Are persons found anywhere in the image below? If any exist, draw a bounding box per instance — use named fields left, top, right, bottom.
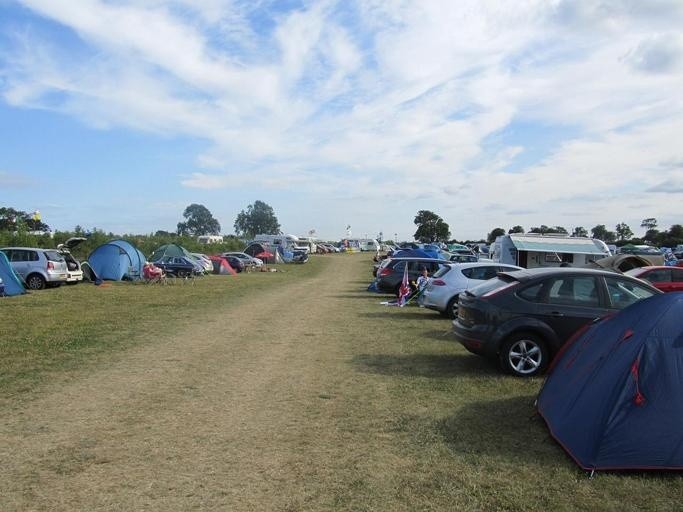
left=413, top=268, right=430, bottom=308
left=144, top=261, right=163, bottom=282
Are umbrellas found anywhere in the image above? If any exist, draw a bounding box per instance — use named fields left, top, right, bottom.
left=255, top=250, right=274, bottom=269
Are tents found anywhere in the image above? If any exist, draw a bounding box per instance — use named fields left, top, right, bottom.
left=533, top=289, right=683, bottom=480
left=368, top=249, right=447, bottom=295
left=209, top=256, right=236, bottom=275
left=87, top=239, right=147, bottom=282
left=0, top=251, right=29, bottom=297
left=147, top=242, right=208, bottom=277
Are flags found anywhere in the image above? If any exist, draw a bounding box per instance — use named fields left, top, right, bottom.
left=380, top=263, right=411, bottom=308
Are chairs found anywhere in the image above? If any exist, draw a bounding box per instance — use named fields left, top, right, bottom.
left=128, top=266, right=160, bottom=286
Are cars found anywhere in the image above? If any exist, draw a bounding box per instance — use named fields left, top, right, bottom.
left=145, top=233, right=340, bottom=279
left=0, top=237, right=88, bottom=289
